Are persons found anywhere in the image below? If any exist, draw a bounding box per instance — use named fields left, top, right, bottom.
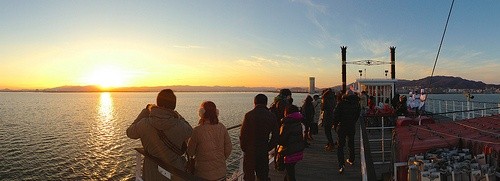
left=420, top=89, right=427, bottom=110
left=187, top=101, right=232, bottom=181
left=414, top=90, right=420, bottom=109
left=312, top=95, right=322, bottom=135
left=320, top=88, right=337, bottom=152
left=300, top=95, right=315, bottom=145
left=277, top=105, right=305, bottom=181
left=268, top=95, right=280, bottom=114
left=126, top=89, right=193, bottom=181
left=333, top=89, right=361, bottom=172
left=358, top=91, right=368, bottom=108
left=368, top=95, right=374, bottom=108
left=335, top=90, right=346, bottom=102
left=409, top=91, right=414, bottom=108
left=271, top=89, right=294, bottom=173
left=240, top=94, right=280, bottom=181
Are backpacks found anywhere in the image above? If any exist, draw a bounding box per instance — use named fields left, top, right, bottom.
left=268, top=98, right=281, bottom=122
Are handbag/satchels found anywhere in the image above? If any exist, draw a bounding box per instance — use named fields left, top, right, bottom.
left=187, top=154, right=195, bottom=175
left=310, top=121, right=318, bottom=134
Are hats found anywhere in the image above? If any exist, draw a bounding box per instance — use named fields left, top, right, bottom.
left=305, top=96, right=313, bottom=101
left=280, top=89, right=292, bottom=97
left=157, top=89, right=176, bottom=107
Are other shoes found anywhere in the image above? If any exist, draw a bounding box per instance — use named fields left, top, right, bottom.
left=274, top=167, right=287, bottom=174
left=346, top=158, right=354, bottom=165
left=324, top=145, right=334, bottom=151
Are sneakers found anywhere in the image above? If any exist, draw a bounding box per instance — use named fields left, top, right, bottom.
left=340, top=168, right=344, bottom=173
